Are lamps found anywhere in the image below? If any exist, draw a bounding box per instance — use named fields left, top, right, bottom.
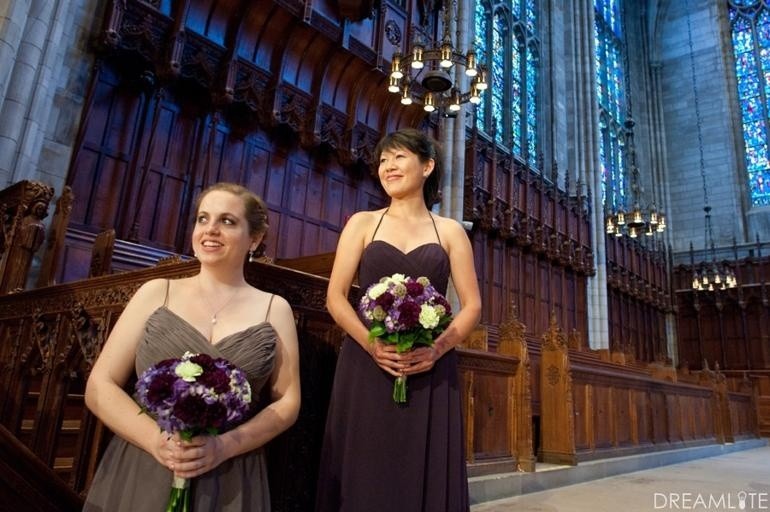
left=388, top=2, right=489, bottom=114
left=603, top=2, right=739, bottom=292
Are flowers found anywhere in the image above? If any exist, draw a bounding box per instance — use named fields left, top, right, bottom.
left=137, top=350, right=252, bottom=511
left=359, top=272, right=450, bottom=405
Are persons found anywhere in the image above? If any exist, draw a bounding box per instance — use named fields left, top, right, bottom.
left=78, top=179, right=304, bottom=510
left=312, top=125, right=486, bottom=512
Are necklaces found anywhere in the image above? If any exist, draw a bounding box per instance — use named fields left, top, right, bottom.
left=194, top=274, right=244, bottom=329
